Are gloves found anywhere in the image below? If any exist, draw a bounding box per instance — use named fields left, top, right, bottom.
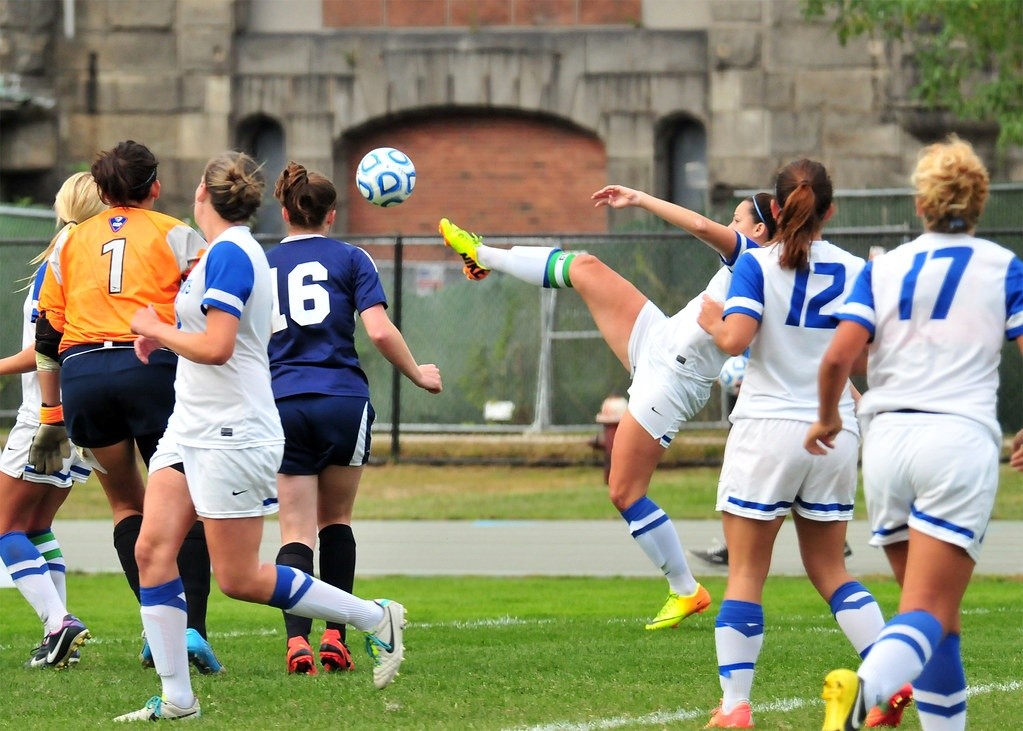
left=28, top=405, right=71, bottom=476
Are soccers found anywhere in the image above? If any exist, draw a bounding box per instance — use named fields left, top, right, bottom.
left=356, top=147, right=417, bottom=208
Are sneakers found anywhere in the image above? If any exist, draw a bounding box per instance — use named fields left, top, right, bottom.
left=138, top=629, right=156, bottom=669
left=29, top=633, right=81, bottom=669
left=285, top=635, right=319, bottom=675
left=818, top=668, right=867, bottom=731
left=703, top=698, right=755, bottom=728
left=319, top=628, right=356, bottom=673
left=44, top=613, right=91, bottom=671
left=437, top=218, right=491, bottom=281
left=362, top=598, right=409, bottom=690
left=113, top=695, right=202, bottom=726
left=865, top=684, right=915, bottom=729
left=645, top=582, right=711, bottom=630
left=186, top=627, right=226, bottom=675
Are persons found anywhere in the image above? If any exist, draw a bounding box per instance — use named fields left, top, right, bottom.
left=439, top=185, right=778, bottom=629
left=697, top=159, right=912, bottom=731
left=802, top=143, right=1023, bottom=731
left=28, top=140, right=225, bottom=675
left=115, top=152, right=410, bottom=722
left=0, top=173, right=110, bottom=672
left=264, top=161, right=442, bottom=674
left=693, top=350, right=862, bottom=564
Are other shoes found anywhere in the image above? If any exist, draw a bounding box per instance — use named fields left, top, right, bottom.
left=694, top=547, right=728, bottom=567
